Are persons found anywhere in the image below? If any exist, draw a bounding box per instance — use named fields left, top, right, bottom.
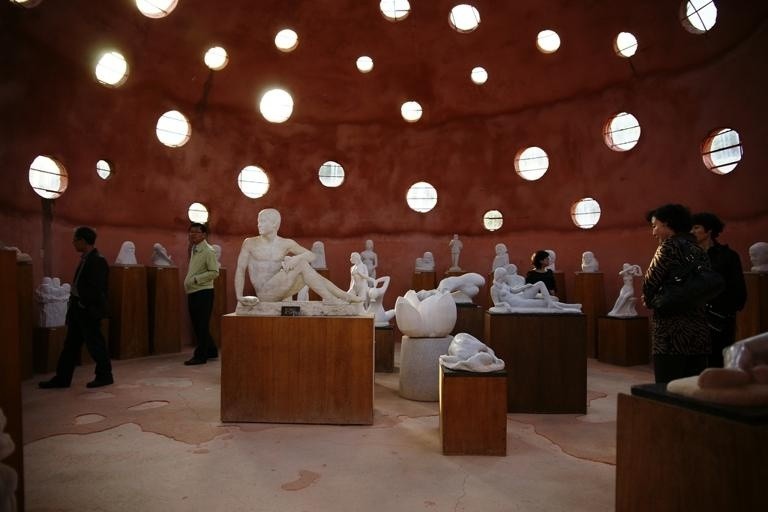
left=38, top=224, right=115, bottom=389
left=416, top=252, right=435, bottom=271
left=607, top=263, right=643, bottom=317
left=234, top=208, right=364, bottom=306
left=545, top=250, right=556, bottom=272
left=749, top=242, right=768, bottom=273
left=491, top=265, right=583, bottom=309
left=448, top=234, right=463, bottom=266
left=151, top=243, right=171, bottom=267
left=34, top=277, right=71, bottom=327
left=525, top=250, right=557, bottom=296
left=184, top=223, right=219, bottom=365
left=493, top=243, right=509, bottom=273
left=643, top=203, right=713, bottom=384
left=311, top=241, right=326, bottom=268
left=366, top=276, right=395, bottom=321
left=115, top=241, right=137, bottom=264
left=360, top=240, right=377, bottom=288
left=581, top=251, right=598, bottom=273
left=697, top=333, right=768, bottom=388
left=347, top=252, right=368, bottom=296
left=688, top=211, right=747, bottom=368
left=211, top=244, right=221, bottom=268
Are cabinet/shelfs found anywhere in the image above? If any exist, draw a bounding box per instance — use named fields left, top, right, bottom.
left=598, top=314, right=651, bottom=366
left=735, top=271, right=768, bottom=340
left=451, top=302, right=484, bottom=343
left=574, top=272, right=607, bottom=358
left=615, top=382, right=768, bottom=511
left=445, top=271, right=465, bottom=293
left=110, top=263, right=148, bottom=360
left=17, top=261, right=34, bottom=380
left=439, top=365, right=509, bottom=458
left=413, top=270, right=436, bottom=291
left=219, top=314, right=374, bottom=425
left=375, top=326, right=395, bottom=373
left=1, top=248, right=26, bottom=512
left=147, top=266, right=182, bottom=354
left=553, top=271, right=567, bottom=303
left=210, top=267, right=228, bottom=329
left=483, top=310, right=589, bottom=415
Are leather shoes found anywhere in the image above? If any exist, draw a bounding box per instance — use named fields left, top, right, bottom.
left=38, top=376, right=71, bottom=388
left=207, top=354, right=218, bottom=361
left=86, top=377, right=113, bottom=388
left=184, top=356, right=206, bottom=365
